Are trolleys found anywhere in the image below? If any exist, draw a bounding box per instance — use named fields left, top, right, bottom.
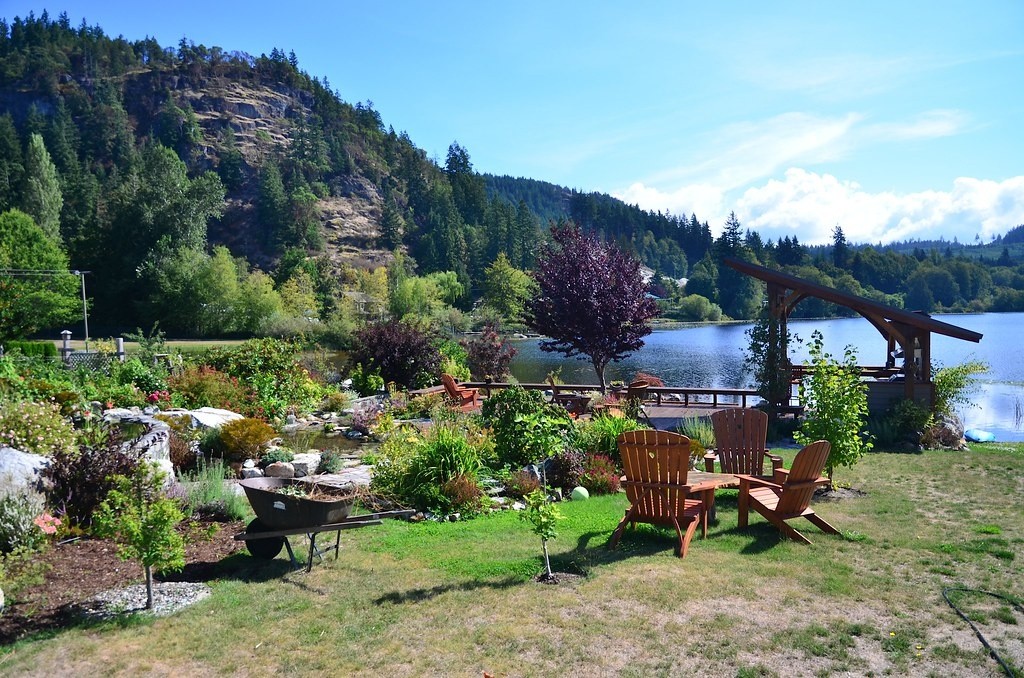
left=234, top=475, right=417, bottom=572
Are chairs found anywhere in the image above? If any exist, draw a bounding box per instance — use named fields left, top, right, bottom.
left=442, top=373, right=480, bottom=407
left=547, top=374, right=580, bottom=416
left=704, top=408, right=783, bottom=519
left=605, top=429, right=708, bottom=559
left=733, top=439, right=843, bottom=547
left=610, top=378, right=650, bottom=420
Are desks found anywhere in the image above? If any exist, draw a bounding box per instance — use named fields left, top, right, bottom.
left=620, top=471, right=756, bottom=518
